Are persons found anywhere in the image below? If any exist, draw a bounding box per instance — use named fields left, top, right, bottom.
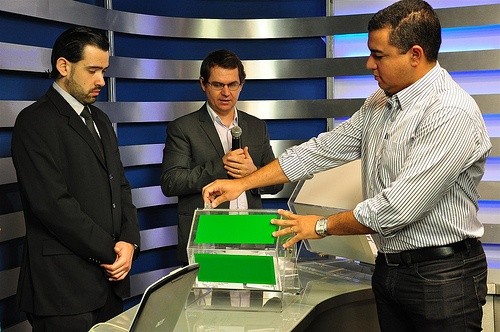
left=200, top=0, right=492, bottom=332
left=161, top=49, right=284, bottom=267
left=11, top=28, right=140, bottom=332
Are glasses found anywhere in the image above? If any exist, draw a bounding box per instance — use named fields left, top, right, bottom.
left=206, top=81, right=240, bottom=91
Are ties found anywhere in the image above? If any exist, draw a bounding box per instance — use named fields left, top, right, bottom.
left=80, top=107, right=104, bottom=162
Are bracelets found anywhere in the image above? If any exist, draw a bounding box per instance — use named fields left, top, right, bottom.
left=132, top=243, right=138, bottom=250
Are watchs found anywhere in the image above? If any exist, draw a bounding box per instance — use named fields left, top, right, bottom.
left=314, top=215, right=336, bottom=238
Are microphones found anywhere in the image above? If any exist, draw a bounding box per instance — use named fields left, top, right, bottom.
left=231, top=126, right=242, bottom=151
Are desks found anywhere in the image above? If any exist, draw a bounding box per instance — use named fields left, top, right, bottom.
left=89, top=259, right=500, bottom=332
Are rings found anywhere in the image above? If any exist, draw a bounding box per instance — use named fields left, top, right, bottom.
left=290, top=225, right=295, bottom=235
left=237, top=169, right=240, bottom=173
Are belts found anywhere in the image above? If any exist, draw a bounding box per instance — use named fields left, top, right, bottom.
left=377, top=238, right=477, bottom=266
